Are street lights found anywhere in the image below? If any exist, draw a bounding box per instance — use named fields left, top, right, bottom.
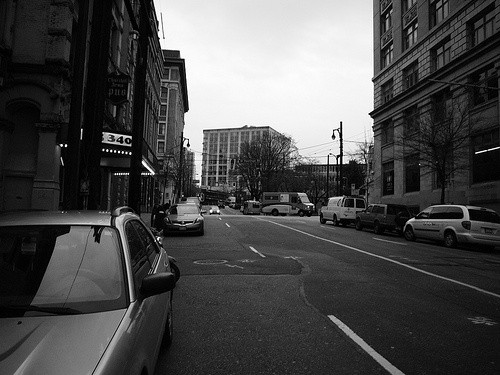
left=178, top=131, right=190, bottom=198
left=332, top=121, right=343, bottom=196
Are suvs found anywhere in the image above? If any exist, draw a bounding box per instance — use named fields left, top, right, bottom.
left=355, top=203, right=420, bottom=237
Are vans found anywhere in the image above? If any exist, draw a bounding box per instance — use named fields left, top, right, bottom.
left=320, top=195, right=368, bottom=227
left=258, top=191, right=316, bottom=217
left=243, top=200, right=261, bottom=216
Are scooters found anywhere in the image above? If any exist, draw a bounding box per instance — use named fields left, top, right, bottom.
left=150, top=225, right=180, bottom=283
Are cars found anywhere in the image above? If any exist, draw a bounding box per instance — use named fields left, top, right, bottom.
left=158, top=202, right=207, bottom=236
left=208, top=205, right=221, bottom=215
left=181, top=196, right=204, bottom=212
left=402, top=204, right=500, bottom=253
left=0, top=205, right=177, bottom=375
